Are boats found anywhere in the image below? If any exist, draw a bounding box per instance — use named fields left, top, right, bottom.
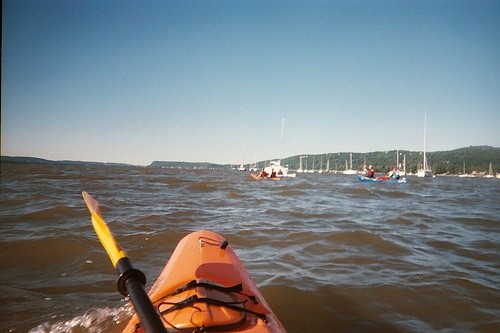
left=250, top=173, right=282, bottom=181
left=356, top=174, right=408, bottom=186
left=460, top=172, right=476, bottom=178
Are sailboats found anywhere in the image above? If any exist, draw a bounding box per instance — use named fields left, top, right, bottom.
left=250, top=154, right=260, bottom=172
left=237, top=139, right=248, bottom=171
left=297, top=151, right=367, bottom=174
left=263, top=116, right=296, bottom=177
left=388, top=150, right=414, bottom=177
left=416, top=113, right=432, bottom=177
left=483, top=160, right=496, bottom=179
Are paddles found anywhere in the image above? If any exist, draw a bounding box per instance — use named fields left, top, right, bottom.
left=81, top=190, right=169, bottom=333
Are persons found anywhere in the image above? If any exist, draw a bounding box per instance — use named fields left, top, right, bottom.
left=278, top=169, right=282, bottom=174
left=390, top=168, right=400, bottom=180
left=365, top=165, right=374, bottom=178
left=270, top=169, right=276, bottom=178
left=260, top=168, right=267, bottom=177
